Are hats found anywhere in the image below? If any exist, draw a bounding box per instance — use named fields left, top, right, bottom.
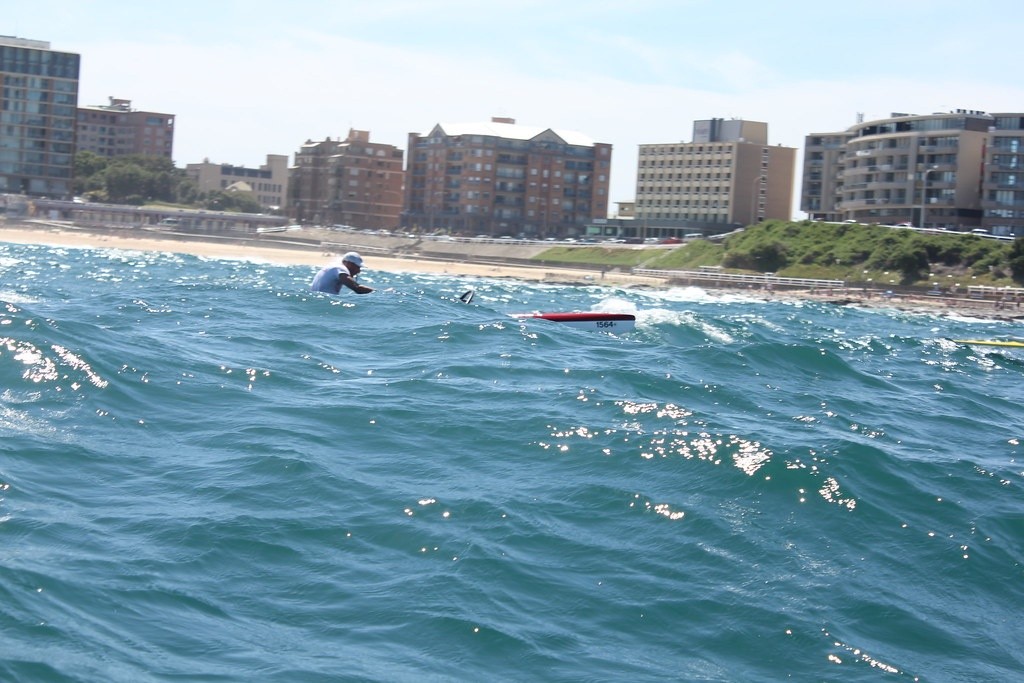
left=342, top=251, right=362, bottom=267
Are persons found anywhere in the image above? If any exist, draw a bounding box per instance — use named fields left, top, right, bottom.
left=780, top=278, right=1023, bottom=312
left=309, top=252, right=373, bottom=297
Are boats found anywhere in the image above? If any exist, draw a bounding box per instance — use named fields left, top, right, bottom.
left=952, top=338, right=1024, bottom=351
left=461, top=292, right=638, bottom=334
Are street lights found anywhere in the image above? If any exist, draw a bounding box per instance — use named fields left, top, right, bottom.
left=919, top=165, right=939, bottom=228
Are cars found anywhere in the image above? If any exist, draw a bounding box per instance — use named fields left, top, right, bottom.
left=810, top=215, right=1017, bottom=241
left=285, top=223, right=704, bottom=246
